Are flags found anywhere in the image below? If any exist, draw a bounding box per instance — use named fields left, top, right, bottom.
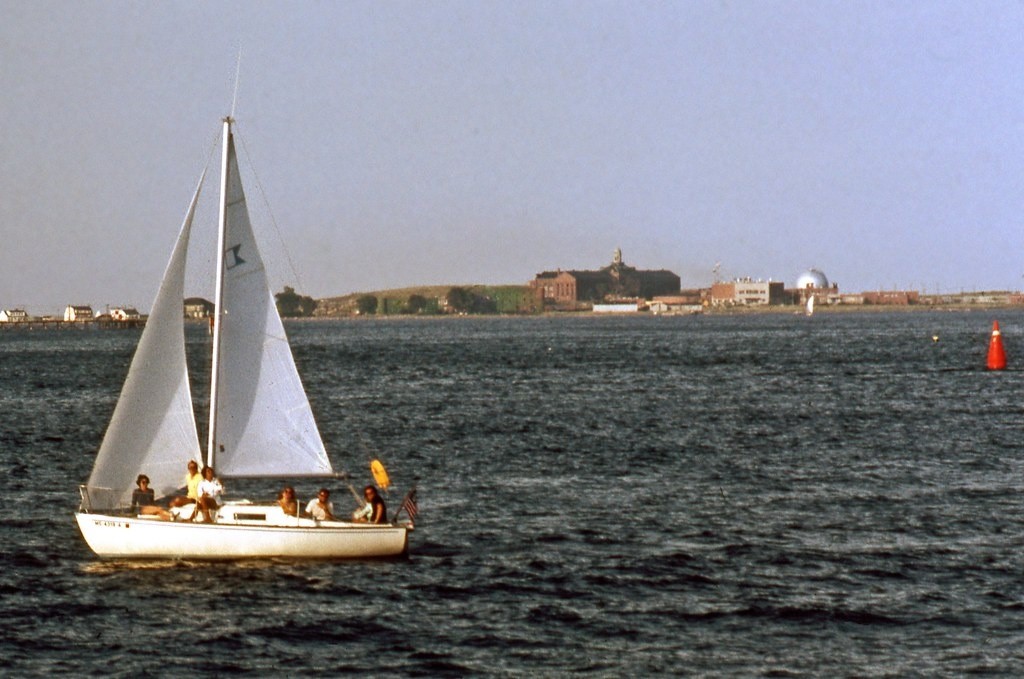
left=404, top=490, right=417, bottom=524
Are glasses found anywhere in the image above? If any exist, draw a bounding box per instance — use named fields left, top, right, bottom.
left=319, top=494, right=328, bottom=498
left=141, top=481, right=147, bottom=484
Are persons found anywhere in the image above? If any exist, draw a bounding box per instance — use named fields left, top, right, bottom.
left=277, top=487, right=297, bottom=515
left=169, top=460, right=204, bottom=507
left=343, top=485, right=387, bottom=524
left=304, top=488, right=338, bottom=520
left=127, top=475, right=180, bottom=522
left=180, top=465, right=224, bottom=523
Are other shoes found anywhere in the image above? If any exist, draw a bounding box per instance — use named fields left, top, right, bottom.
left=202, top=519, right=212, bottom=524
left=174, top=512, right=180, bottom=518
left=169, top=511, right=174, bottom=521
left=182, top=519, right=192, bottom=523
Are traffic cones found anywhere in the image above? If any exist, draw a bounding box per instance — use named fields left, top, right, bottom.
left=987, top=320, right=1008, bottom=371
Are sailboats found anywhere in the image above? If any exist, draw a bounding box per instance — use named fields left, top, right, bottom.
left=68, top=115, right=424, bottom=557
